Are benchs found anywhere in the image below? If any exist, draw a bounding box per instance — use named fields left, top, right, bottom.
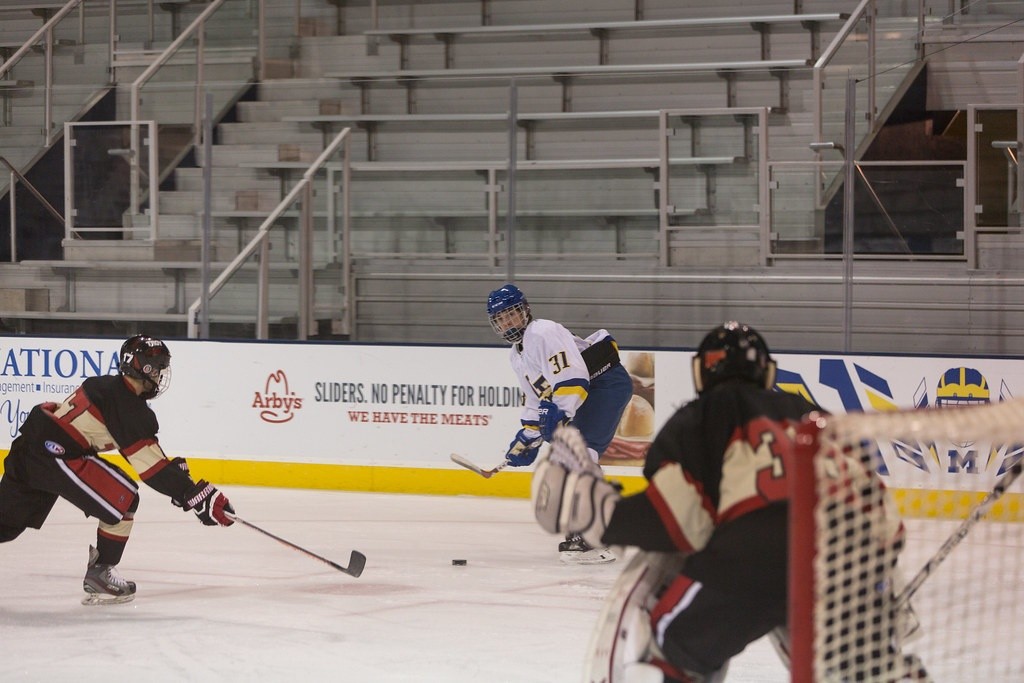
left=0, top=0, right=1024, bottom=356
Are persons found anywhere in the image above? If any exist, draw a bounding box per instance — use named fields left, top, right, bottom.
left=602, top=321, right=930, bottom=683
left=0, top=334, right=235, bottom=605
left=486, top=284, right=633, bottom=564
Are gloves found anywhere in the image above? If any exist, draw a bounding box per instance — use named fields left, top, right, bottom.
left=170, top=456, right=194, bottom=508
left=185, top=478, right=236, bottom=526
left=505, top=428, right=539, bottom=467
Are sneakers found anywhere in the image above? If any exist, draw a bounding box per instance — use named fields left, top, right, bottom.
left=559, top=533, right=616, bottom=567
left=82, top=544, right=136, bottom=605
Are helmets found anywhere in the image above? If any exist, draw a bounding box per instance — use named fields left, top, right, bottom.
left=697, top=321, right=768, bottom=396
left=488, top=283, right=530, bottom=344
left=119, top=335, right=171, bottom=400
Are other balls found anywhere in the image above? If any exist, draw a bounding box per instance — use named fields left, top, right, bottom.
left=451, top=558, right=468, bottom=566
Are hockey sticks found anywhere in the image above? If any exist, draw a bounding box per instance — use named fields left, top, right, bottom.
left=449, top=419, right=568, bottom=480
left=221, top=508, right=367, bottom=579
left=894, top=455, right=1024, bottom=612
